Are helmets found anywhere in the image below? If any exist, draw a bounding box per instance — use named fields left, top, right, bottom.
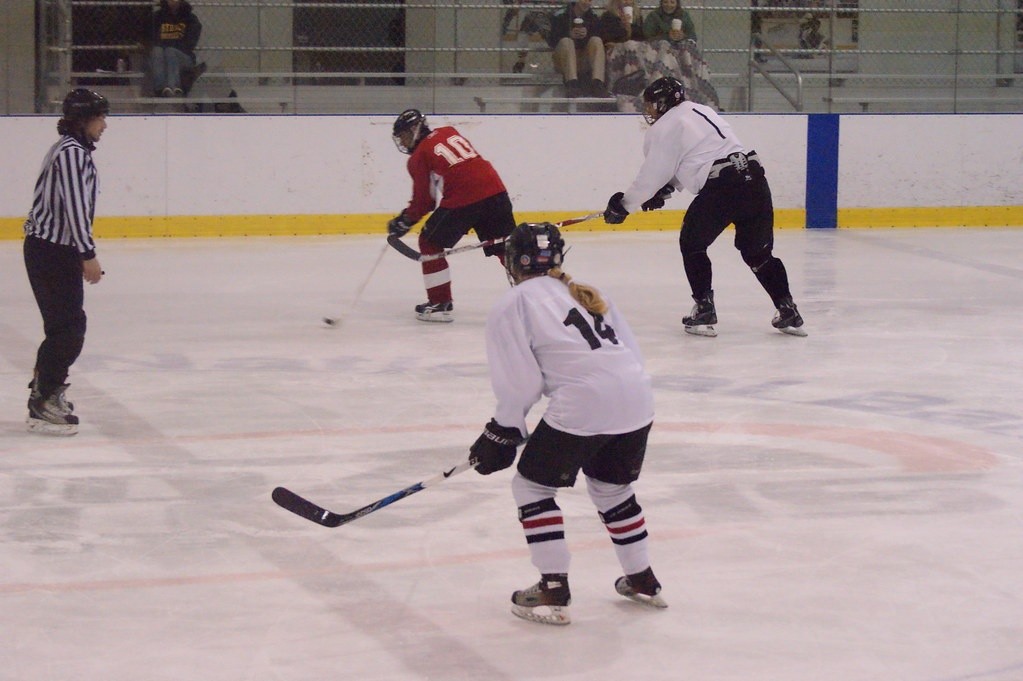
left=61, top=87, right=111, bottom=121
left=504, top=221, right=565, bottom=285
left=642, top=76, right=685, bottom=127
left=391, top=109, right=429, bottom=154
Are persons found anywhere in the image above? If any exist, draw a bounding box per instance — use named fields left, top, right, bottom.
left=21, top=88, right=112, bottom=436
left=467, top=221, right=667, bottom=626
left=144, top=0, right=202, bottom=98
left=379, top=0, right=405, bottom=87
left=604, top=76, right=809, bottom=336
left=293, top=28, right=384, bottom=86
left=116, top=39, right=147, bottom=75
left=499, top=0, right=726, bottom=114
left=751, top=0, right=858, bottom=65
left=384, top=110, right=517, bottom=324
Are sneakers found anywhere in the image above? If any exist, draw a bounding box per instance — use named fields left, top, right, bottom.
left=28, top=368, right=74, bottom=411
left=414, top=297, right=454, bottom=321
left=682, top=290, right=718, bottom=336
left=511, top=572, right=572, bottom=625
left=27, top=378, right=78, bottom=434
left=771, top=295, right=807, bottom=336
left=614, top=568, right=668, bottom=608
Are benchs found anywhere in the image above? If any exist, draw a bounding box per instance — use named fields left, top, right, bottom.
left=44, top=0, right=1023, bottom=112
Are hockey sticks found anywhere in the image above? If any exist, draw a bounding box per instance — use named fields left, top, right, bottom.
left=272, top=432, right=534, bottom=530
left=320, top=242, right=389, bottom=327
left=387, top=194, right=672, bottom=264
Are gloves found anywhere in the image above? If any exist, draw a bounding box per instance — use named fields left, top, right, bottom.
left=641, top=184, right=675, bottom=211
left=468, top=417, right=527, bottom=474
left=604, top=191, right=631, bottom=224
left=387, top=209, right=419, bottom=238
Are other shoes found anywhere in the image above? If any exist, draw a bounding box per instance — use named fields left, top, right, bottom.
left=564, top=78, right=585, bottom=98
left=589, top=80, right=608, bottom=98
left=161, top=87, right=173, bottom=97
left=173, top=87, right=184, bottom=97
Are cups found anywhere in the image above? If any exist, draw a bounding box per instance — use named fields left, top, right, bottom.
left=672, top=19, right=682, bottom=31
left=573, top=18, right=584, bottom=28
left=623, top=6, right=633, bottom=23
